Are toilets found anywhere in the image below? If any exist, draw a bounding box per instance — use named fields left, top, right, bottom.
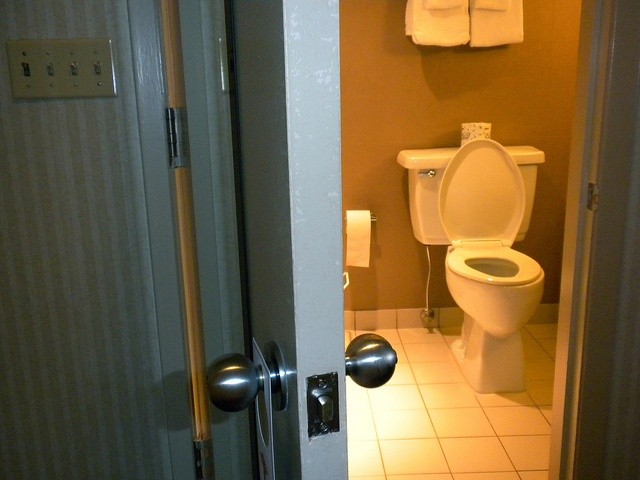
left=397, top=142, right=548, bottom=395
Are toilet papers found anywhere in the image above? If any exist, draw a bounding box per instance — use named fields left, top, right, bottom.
left=346, top=208, right=372, bottom=270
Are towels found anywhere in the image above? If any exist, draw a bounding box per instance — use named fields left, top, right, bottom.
left=470, top=0, right=524, bottom=48
left=405, top=0, right=470, bottom=48
